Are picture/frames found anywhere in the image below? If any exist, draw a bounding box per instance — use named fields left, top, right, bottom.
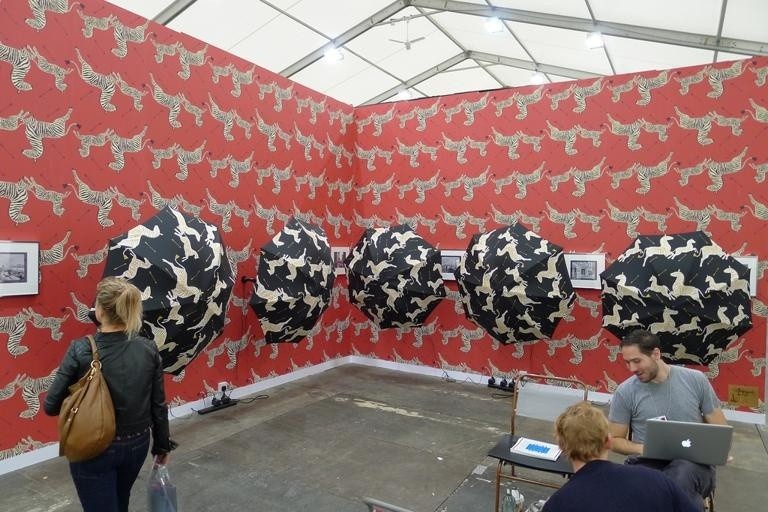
left=330, top=246, right=349, bottom=275
left=438, top=250, right=466, bottom=281
left=732, top=255, right=758, bottom=298
left=562, top=253, right=605, bottom=290
left=0, top=240, right=41, bottom=296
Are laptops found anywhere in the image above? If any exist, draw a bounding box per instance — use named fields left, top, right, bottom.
left=637, top=419, right=734, bottom=469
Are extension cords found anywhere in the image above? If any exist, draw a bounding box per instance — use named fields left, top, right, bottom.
left=487, top=381, right=515, bottom=394
left=197, top=399, right=236, bottom=415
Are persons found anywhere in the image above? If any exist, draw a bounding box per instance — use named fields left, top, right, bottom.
left=606, top=327, right=734, bottom=512
left=538, top=400, right=706, bottom=512
left=42, top=278, right=178, bottom=512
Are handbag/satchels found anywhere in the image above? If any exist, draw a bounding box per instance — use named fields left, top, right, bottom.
left=143, top=453, right=181, bottom=511
left=56, top=327, right=115, bottom=464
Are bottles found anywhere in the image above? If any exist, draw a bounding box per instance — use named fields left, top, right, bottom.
left=501, top=486, right=522, bottom=512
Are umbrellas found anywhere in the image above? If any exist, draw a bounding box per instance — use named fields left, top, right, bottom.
left=452, top=220, right=578, bottom=346
left=86, top=205, right=235, bottom=377
left=596, top=230, right=754, bottom=369
left=244, top=214, right=335, bottom=345
left=342, top=221, right=447, bottom=330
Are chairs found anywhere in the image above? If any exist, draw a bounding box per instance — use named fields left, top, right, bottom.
left=360, top=496, right=412, bottom=512
left=486, top=373, right=588, bottom=512
left=628, top=414, right=715, bottom=512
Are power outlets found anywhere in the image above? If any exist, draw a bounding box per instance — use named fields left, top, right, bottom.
left=218, top=381, right=228, bottom=392
